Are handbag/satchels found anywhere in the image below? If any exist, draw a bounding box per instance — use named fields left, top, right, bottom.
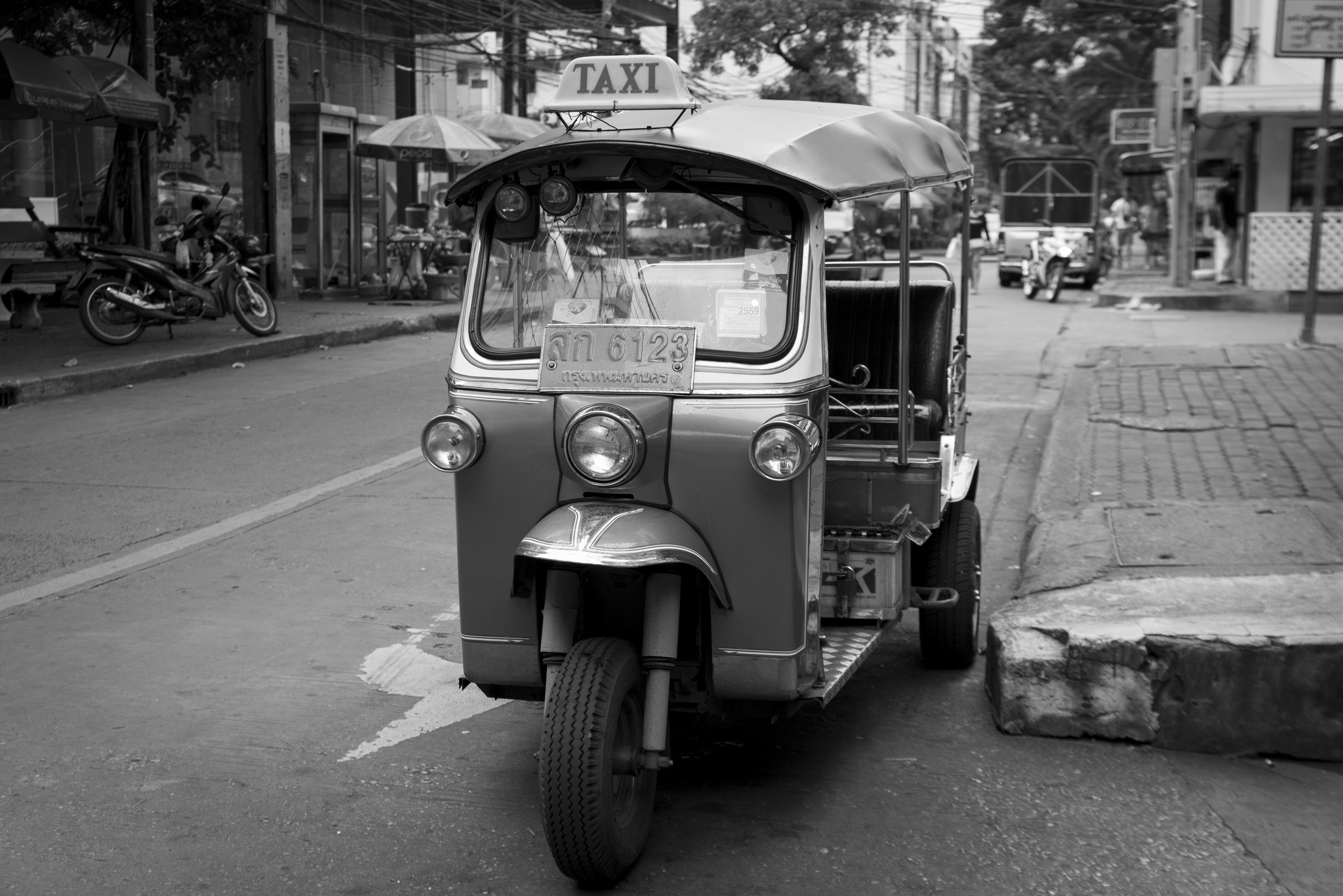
left=174, top=237, right=191, bottom=272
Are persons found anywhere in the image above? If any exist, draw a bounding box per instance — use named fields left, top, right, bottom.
left=958, top=195, right=992, bottom=295
left=1101, top=165, right=1246, bottom=285
left=183, top=193, right=212, bottom=264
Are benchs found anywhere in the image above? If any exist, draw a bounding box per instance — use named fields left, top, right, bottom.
left=823, top=281, right=956, bottom=494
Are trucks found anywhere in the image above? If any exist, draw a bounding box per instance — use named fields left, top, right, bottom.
left=997, top=154, right=1102, bottom=287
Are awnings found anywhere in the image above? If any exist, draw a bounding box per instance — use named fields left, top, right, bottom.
left=1182, top=113, right=1255, bottom=182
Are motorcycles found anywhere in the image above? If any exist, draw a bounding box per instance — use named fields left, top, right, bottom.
left=73, top=181, right=279, bottom=346
left=419, top=53, right=987, bottom=893
left=1018, top=227, right=1076, bottom=303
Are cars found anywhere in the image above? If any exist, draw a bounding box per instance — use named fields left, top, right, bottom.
left=820, top=206, right=889, bottom=285
left=78, top=168, right=244, bottom=236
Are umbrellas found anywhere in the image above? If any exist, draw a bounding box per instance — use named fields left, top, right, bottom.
left=1, top=41, right=171, bottom=247
left=882, top=191, right=934, bottom=212
left=353, top=112, right=499, bottom=236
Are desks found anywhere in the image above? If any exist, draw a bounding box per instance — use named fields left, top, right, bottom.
left=386, top=235, right=462, bottom=300
